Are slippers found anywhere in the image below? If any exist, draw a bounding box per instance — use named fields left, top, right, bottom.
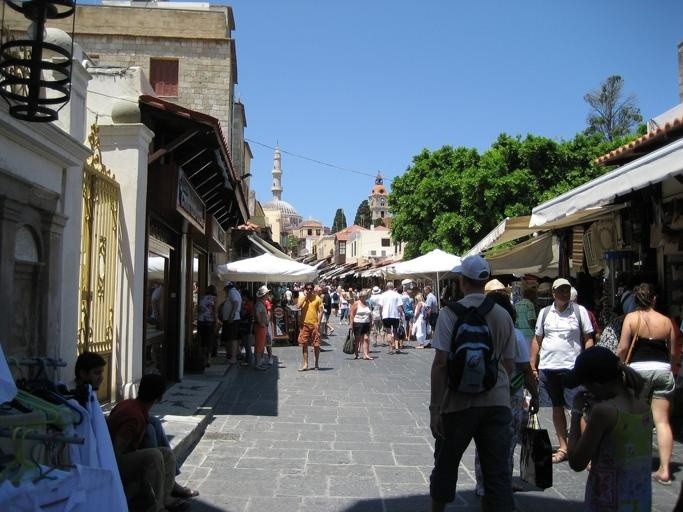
left=170, top=482, right=198, bottom=498
left=553, top=449, right=673, bottom=486
left=165, top=498, right=190, bottom=510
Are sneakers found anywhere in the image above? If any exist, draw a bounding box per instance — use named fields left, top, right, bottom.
left=228, top=357, right=374, bottom=372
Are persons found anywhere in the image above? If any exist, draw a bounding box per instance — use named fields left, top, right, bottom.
left=486, top=279, right=513, bottom=318
left=475, top=292, right=538, bottom=501
left=617, top=283, right=680, bottom=485
left=199, top=281, right=324, bottom=371
left=348, top=278, right=463, bottom=360
left=106, top=374, right=198, bottom=512
left=566, top=286, right=600, bottom=437
left=430, top=256, right=516, bottom=512
left=515, top=283, right=538, bottom=344
left=66, top=351, right=107, bottom=390
left=568, top=347, right=653, bottom=512
left=317, top=281, right=357, bottom=336
left=619, top=275, right=637, bottom=315
left=529, top=278, right=594, bottom=471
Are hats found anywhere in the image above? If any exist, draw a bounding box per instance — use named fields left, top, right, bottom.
left=552, top=278, right=571, bottom=289
left=372, top=286, right=381, bottom=294
left=223, top=280, right=232, bottom=287
left=256, top=285, right=271, bottom=298
left=452, top=255, right=490, bottom=280
left=484, top=279, right=511, bottom=298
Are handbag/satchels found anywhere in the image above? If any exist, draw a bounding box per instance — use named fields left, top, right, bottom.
left=342, top=330, right=355, bottom=354
left=519, top=412, right=552, bottom=489
left=395, top=326, right=407, bottom=340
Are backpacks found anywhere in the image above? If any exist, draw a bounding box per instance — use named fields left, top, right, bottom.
left=423, top=307, right=430, bottom=319
left=441, top=295, right=501, bottom=396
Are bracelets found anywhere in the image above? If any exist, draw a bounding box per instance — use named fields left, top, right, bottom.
left=570, top=408, right=581, bottom=416
left=429, top=405, right=440, bottom=413
